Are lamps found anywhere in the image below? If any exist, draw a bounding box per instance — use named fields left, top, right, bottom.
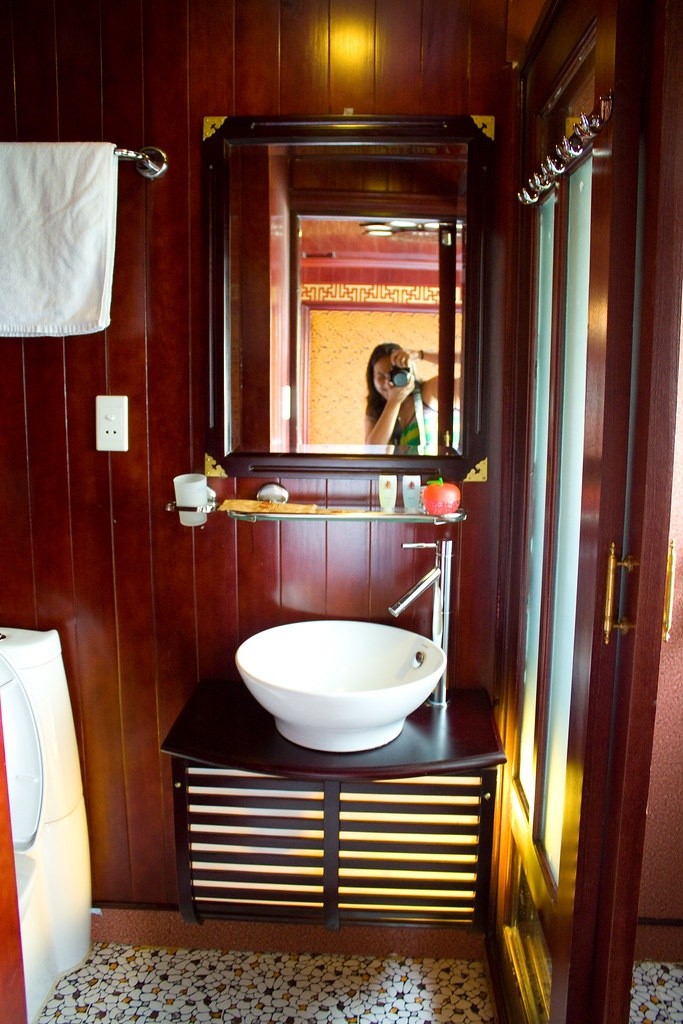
left=358, top=217, right=469, bottom=244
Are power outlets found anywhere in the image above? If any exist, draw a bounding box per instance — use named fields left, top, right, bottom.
left=282, top=386, right=291, bottom=422
left=95, top=394, right=130, bottom=453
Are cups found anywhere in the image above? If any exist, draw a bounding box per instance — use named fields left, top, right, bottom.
left=174, top=473, right=208, bottom=527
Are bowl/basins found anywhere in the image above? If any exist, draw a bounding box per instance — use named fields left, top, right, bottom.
left=235, top=620, right=445, bottom=751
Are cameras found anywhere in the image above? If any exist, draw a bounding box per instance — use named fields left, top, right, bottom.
left=389, top=364, right=411, bottom=387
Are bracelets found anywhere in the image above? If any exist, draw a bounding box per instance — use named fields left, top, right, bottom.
left=421, top=350, right=424, bottom=360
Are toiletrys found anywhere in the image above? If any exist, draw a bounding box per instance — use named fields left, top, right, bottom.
left=379, top=475, right=397, bottom=513
left=402, top=475, right=421, bottom=512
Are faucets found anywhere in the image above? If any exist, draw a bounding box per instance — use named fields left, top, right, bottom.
left=388, top=538, right=452, bottom=708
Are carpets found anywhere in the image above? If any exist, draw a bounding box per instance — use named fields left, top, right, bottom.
left=33, top=940, right=497, bottom=1024
left=625, top=961, right=683, bottom=1024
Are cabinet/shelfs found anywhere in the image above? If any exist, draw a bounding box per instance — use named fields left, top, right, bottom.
left=158, top=678, right=507, bottom=937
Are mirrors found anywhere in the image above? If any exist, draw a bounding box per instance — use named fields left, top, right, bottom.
left=201, top=111, right=491, bottom=484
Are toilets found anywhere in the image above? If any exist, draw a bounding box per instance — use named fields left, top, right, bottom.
left=1, top=627, right=91, bottom=1024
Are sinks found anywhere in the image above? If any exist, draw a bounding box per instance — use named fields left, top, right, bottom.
left=236, top=620, right=445, bottom=753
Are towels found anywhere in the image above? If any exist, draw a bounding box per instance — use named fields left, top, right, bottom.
left=0, top=141, right=118, bottom=337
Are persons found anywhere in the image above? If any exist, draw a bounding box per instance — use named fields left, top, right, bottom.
left=365, top=342, right=461, bottom=455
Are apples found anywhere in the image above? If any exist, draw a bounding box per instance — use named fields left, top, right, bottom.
left=423, top=476, right=461, bottom=516
